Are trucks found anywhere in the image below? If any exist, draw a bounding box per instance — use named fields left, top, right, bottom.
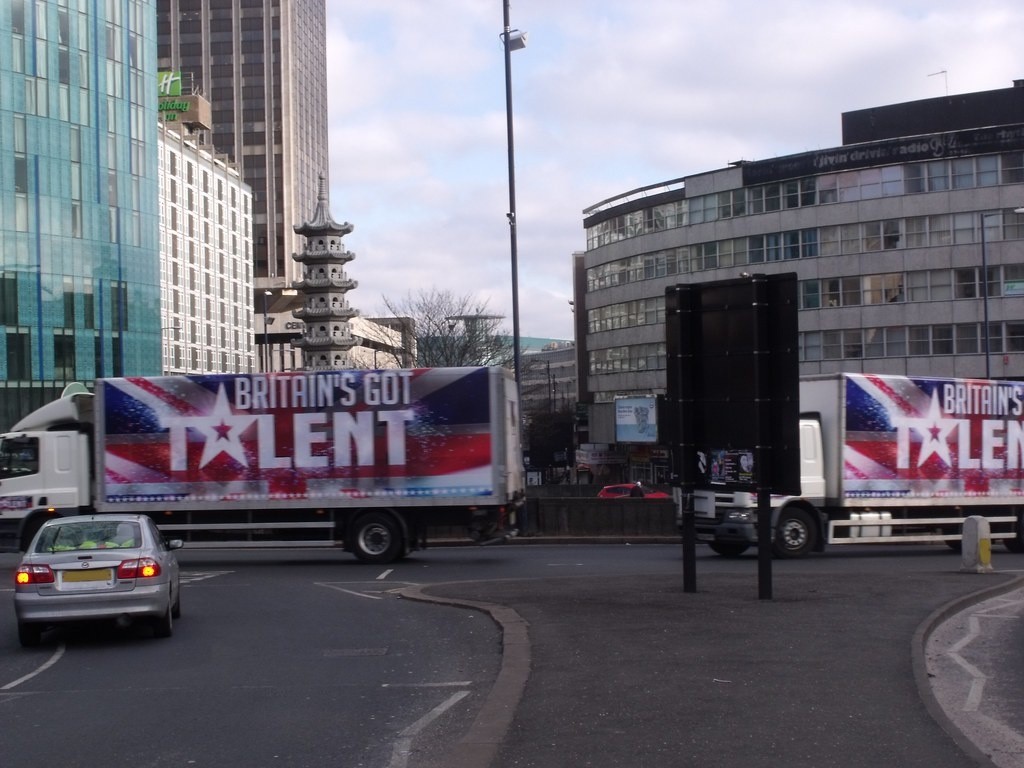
left=0, top=366, right=526, bottom=562
left=672, top=372, right=1024, bottom=555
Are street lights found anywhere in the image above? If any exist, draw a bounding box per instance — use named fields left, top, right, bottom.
left=501, top=26, right=530, bottom=377
left=262, top=291, right=274, bottom=371
left=533, top=359, right=556, bottom=412
left=982, top=208, right=1024, bottom=379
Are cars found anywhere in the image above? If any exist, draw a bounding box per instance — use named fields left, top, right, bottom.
left=597, top=485, right=670, bottom=498
left=14, top=514, right=184, bottom=639
